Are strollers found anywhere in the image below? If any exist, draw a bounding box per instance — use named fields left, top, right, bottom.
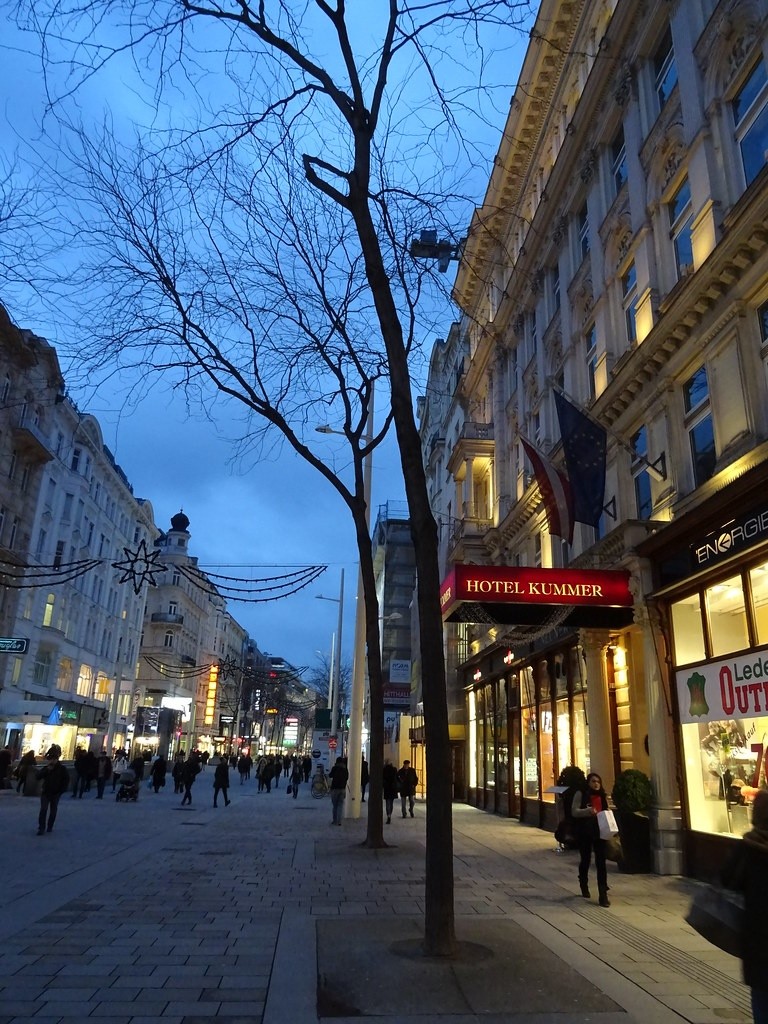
left=115, top=769, right=139, bottom=803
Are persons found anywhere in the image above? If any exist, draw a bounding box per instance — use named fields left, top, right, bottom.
left=71, top=746, right=130, bottom=800
left=397, top=760, right=418, bottom=818
left=172, top=749, right=209, bottom=805
left=34, top=744, right=69, bottom=835
left=255, top=753, right=312, bottom=798
left=384, top=759, right=400, bottom=824
left=238, top=754, right=253, bottom=784
left=150, top=754, right=167, bottom=793
left=329, top=757, right=348, bottom=826
left=0, top=745, right=11, bottom=777
left=213, top=757, right=231, bottom=808
left=129, top=748, right=151, bottom=780
left=741, top=789, right=768, bottom=1024
left=361, top=756, right=369, bottom=801
left=16, top=750, right=37, bottom=793
left=230, top=753, right=238, bottom=770
left=572, top=773, right=611, bottom=905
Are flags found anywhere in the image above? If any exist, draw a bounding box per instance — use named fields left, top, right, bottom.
left=519, top=436, right=574, bottom=547
left=552, top=387, right=608, bottom=526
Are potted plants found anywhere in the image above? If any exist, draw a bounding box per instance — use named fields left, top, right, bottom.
left=556, top=764, right=585, bottom=818
left=611, top=768, right=655, bottom=872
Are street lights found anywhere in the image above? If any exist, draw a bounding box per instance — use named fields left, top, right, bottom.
left=314, top=567, right=346, bottom=788
left=314, top=376, right=375, bottom=822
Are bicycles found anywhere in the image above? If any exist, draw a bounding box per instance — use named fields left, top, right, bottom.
left=311, top=763, right=333, bottom=799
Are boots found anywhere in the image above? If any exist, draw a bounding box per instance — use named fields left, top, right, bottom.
left=579, top=877, right=591, bottom=897
left=599, top=887, right=611, bottom=907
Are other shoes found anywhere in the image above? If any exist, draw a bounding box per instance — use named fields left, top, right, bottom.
left=386, top=817, right=391, bottom=824
left=409, top=810, right=415, bottom=818
left=404, top=812, right=407, bottom=818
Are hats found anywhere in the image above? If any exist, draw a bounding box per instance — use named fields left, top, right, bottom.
left=403, top=760, right=410, bottom=765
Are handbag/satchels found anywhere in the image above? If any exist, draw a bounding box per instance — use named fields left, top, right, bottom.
left=255, top=774, right=261, bottom=778
left=148, top=775, right=153, bottom=790
left=162, top=777, right=165, bottom=787
left=597, top=807, right=619, bottom=840
left=686, top=882, right=745, bottom=959
left=287, top=781, right=292, bottom=794
